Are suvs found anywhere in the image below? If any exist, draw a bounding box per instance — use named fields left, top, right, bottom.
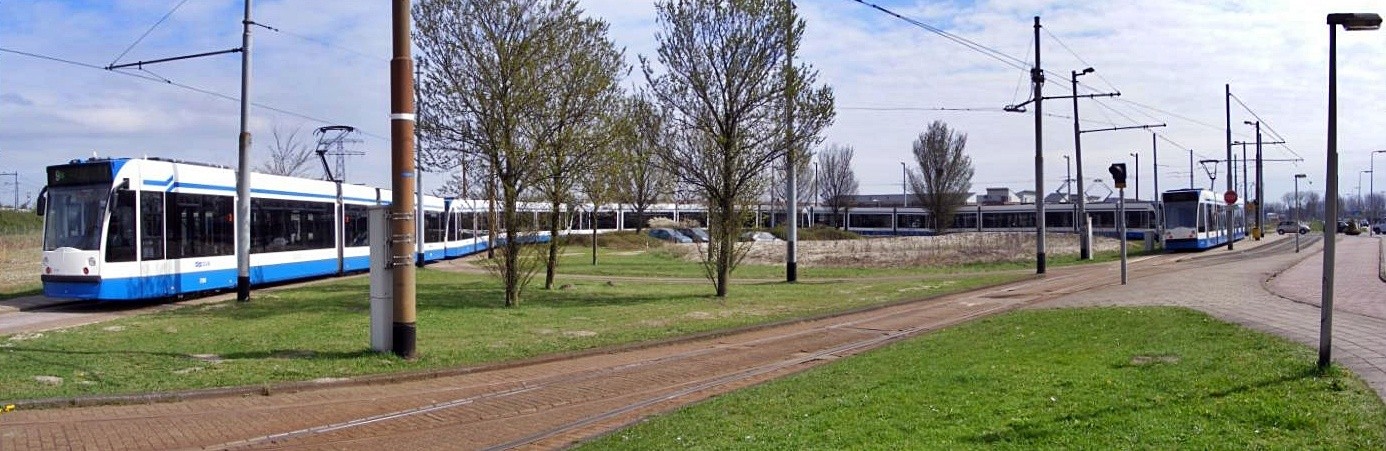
left=1276, top=220, right=1311, bottom=235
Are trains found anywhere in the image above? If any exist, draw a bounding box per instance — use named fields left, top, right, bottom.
left=1159, top=188, right=1246, bottom=252
left=36, top=157, right=1157, bottom=303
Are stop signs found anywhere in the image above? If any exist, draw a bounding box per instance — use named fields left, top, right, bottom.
left=1224, top=191, right=1237, bottom=204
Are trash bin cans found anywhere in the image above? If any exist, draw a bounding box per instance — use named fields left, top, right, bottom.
left=1252, top=228, right=1260, bottom=238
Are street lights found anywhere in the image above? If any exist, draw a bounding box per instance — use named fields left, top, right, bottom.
left=812, top=161, right=818, bottom=206
left=1130, top=152, right=1138, bottom=201
left=1234, top=120, right=1261, bottom=241
left=1316, top=12, right=1383, bottom=369
left=1072, top=67, right=1096, bottom=259
left=900, top=161, right=906, bottom=208
left=1294, top=174, right=1307, bottom=253
left=1063, top=155, right=1071, bottom=204
left=1358, top=150, right=1386, bottom=236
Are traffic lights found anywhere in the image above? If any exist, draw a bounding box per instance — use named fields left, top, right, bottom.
left=1108, top=163, right=1126, bottom=179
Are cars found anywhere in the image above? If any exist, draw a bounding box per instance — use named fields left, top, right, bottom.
left=675, top=227, right=709, bottom=243
left=740, top=231, right=783, bottom=242
left=1321, top=218, right=1369, bottom=233
left=1372, top=217, right=1386, bottom=233
left=647, top=227, right=694, bottom=244
